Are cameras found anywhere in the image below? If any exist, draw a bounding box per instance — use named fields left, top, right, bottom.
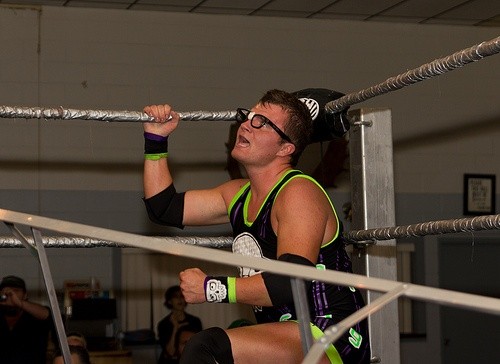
left=0, top=293, right=8, bottom=302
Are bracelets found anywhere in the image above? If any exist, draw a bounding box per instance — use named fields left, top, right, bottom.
left=226, top=277, right=237, bottom=304
left=145, top=131, right=169, bottom=160
left=204, top=276, right=229, bottom=303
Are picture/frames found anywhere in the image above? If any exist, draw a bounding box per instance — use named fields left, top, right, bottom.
left=463, top=173, right=496, bottom=217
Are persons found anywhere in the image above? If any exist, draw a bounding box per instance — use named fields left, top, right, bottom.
left=0, top=275, right=255, bottom=364
left=143, top=89, right=371, bottom=363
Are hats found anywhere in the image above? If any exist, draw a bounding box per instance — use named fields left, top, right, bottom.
left=0, top=276, right=25, bottom=290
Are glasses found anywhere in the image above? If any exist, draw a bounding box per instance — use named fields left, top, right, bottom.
left=236, top=108, right=293, bottom=143
